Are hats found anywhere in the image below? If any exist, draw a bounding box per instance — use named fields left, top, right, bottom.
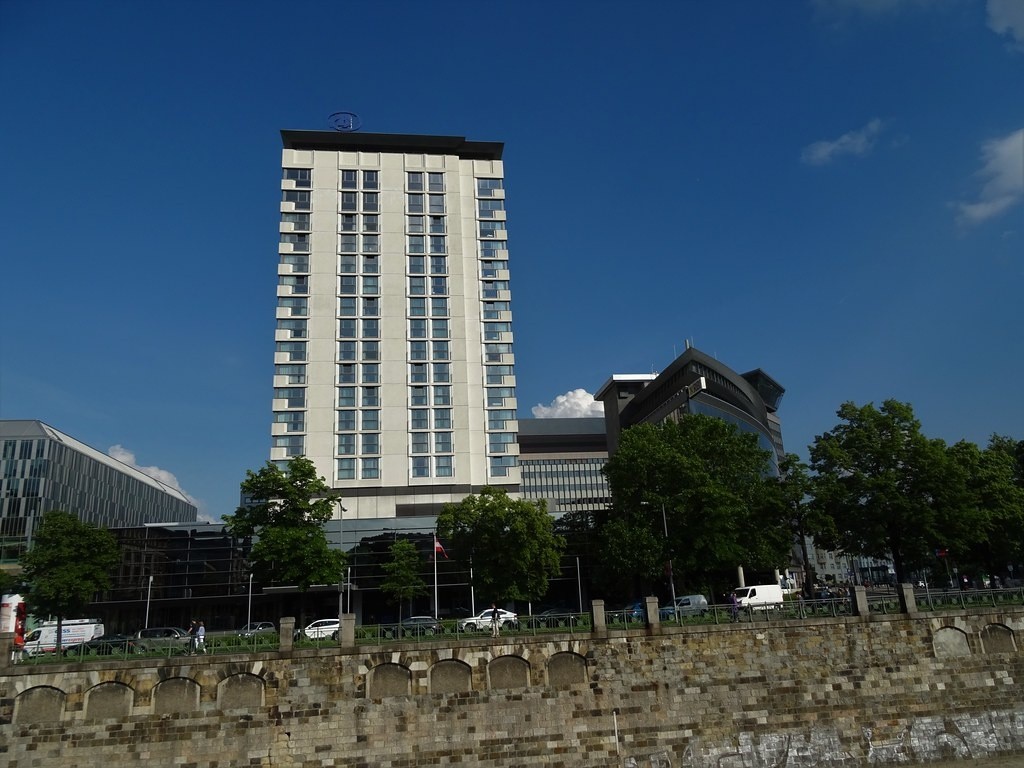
left=199, top=621, right=204, bottom=626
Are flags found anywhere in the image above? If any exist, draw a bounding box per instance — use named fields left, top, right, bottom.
left=436, top=539, right=448, bottom=558
left=931, top=543, right=946, bottom=566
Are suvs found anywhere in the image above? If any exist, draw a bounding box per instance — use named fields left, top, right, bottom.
left=862, top=579, right=876, bottom=587
left=457, top=609, right=518, bottom=632
left=131, top=628, right=207, bottom=653
left=916, top=581, right=929, bottom=589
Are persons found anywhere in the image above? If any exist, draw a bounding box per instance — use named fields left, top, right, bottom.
left=959, top=574, right=968, bottom=591
left=836, top=587, right=850, bottom=615
left=12, top=619, right=26, bottom=664
left=864, top=582, right=869, bottom=587
left=794, top=592, right=808, bottom=618
left=729, top=593, right=739, bottom=623
left=185, top=618, right=207, bottom=656
left=491, top=603, right=500, bottom=638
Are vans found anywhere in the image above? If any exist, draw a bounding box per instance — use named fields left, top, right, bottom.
left=12, top=619, right=104, bottom=656
left=660, top=594, right=709, bottom=619
left=731, top=585, right=784, bottom=612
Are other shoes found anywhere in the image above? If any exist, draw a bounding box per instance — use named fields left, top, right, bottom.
left=21, top=660, right=24, bottom=663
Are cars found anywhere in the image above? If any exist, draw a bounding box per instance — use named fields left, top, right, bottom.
left=527, top=608, right=580, bottom=629
left=383, top=617, right=445, bottom=638
left=294, top=619, right=340, bottom=643
left=620, top=602, right=678, bottom=623
left=805, top=588, right=841, bottom=608
left=238, top=622, right=276, bottom=638
left=61, top=633, right=137, bottom=654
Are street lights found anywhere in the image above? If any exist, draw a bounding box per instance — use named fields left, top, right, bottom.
left=336, top=495, right=348, bottom=615
left=249, top=574, right=254, bottom=633
left=145, top=575, right=154, bottom=627
